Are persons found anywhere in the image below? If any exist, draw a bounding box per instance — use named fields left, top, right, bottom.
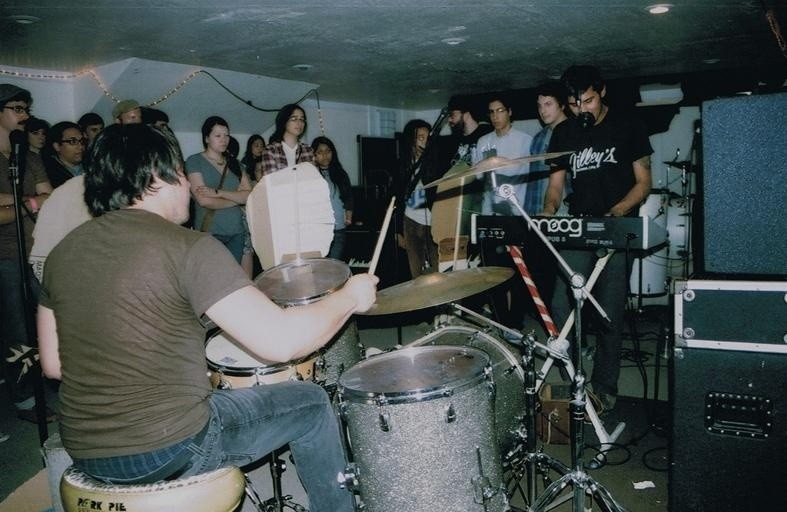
left=37, top=126, right=379, bottom=512
left=390, top=66, right=653, bottom=411
left=1, top=84, right=353, bottom=440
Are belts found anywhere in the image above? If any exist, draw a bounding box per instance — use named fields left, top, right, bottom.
left=86, top=415, right=211, bottom=485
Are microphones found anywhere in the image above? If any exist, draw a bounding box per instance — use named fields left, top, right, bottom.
left=10, top=130, right=26, bottom=183
left=496, top=183, right=520, bottom=206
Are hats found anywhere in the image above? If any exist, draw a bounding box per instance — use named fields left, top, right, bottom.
left=0, top=83, right=30, bottom=103
left=112, top=99, right=147, bottom=121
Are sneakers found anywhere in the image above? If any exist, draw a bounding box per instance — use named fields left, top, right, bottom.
left=14, top=404, right=59, bottom=426
left=501, top=326, right=525, bottom=344
left=582, top=388, right=619, bottom=426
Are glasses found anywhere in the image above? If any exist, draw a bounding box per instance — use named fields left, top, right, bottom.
left=59, top=138, right=86, bottom=146
left=289, top=116, right=305, bottom=122
left=1, top=105, right=29, bottom=113
left=487, top=109, right=509, bottom=115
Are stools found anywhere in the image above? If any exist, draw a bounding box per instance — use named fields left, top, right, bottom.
left=59, top=465, right=247, bottom=511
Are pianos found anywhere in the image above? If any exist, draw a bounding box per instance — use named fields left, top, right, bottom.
left=470, top=211, right=669, bottom=250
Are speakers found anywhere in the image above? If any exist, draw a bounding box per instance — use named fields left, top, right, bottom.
left=670, top=349, right=787, bottom=512
left=429, top=107, right=449, bottom=138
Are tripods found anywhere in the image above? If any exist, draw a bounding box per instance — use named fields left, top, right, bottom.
left=452, top=288, right=631, bottom=512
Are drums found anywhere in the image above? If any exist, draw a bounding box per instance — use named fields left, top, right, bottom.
left=204, top=329, right=316, bottom=390
left=255, top=258, right=362, bottom=390
left=337, top=326, right=526, bottom=510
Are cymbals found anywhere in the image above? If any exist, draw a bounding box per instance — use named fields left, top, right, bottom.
left=362, top=265, right=516, bottom=317
left=422, top=151, right=573, bottom=189
